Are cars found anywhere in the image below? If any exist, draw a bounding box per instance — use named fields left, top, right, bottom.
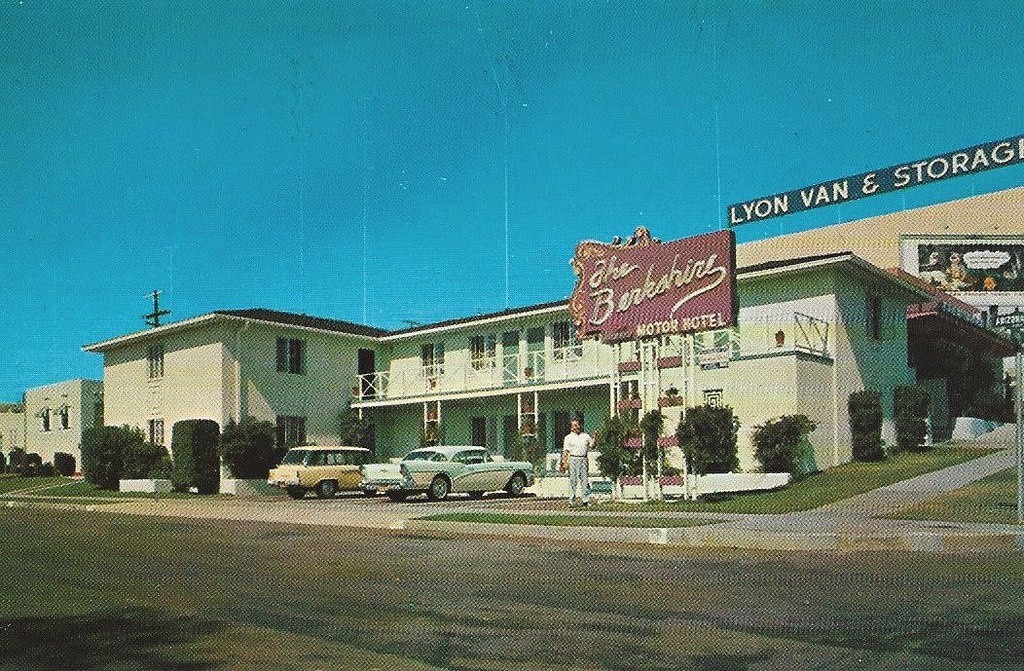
left=360, top=444, right=536, bottom=501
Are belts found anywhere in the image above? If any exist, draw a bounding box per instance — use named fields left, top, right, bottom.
left=570, top=454, right=587, bottom=457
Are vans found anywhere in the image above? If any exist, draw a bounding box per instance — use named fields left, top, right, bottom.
left=268, top=445, right=379, bottom=498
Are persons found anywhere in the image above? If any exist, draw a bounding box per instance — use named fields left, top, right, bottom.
left=561, top=417, right=597, bottom=509
left=921, top=250, right=968, bottom=291
